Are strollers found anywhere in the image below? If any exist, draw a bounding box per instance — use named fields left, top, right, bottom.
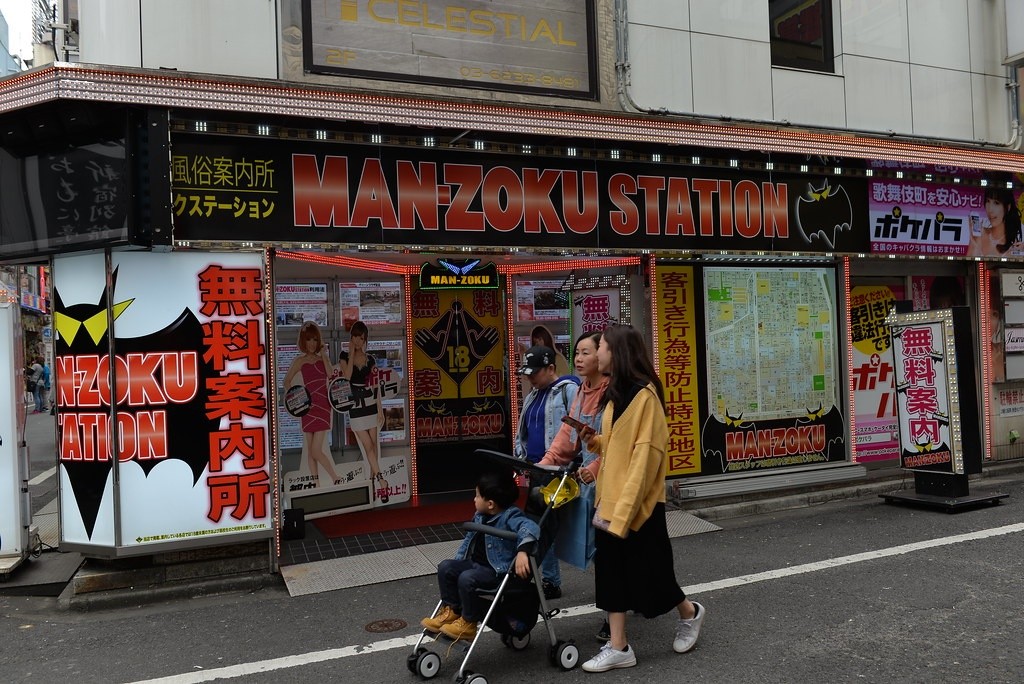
left=407, top=448, right=582, bottom=684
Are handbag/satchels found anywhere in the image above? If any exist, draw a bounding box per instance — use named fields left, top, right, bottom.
left=554, top=469, right=597, bottom=570
left=27, top=381, right=36, bottom=391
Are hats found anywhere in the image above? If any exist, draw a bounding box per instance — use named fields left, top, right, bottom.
left=514, top=346, right=555, bottom=375
left=541, top=476, right=579, bottom=508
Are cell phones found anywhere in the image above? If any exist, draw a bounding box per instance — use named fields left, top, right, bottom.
left=560, top=415, right=596, bottom=438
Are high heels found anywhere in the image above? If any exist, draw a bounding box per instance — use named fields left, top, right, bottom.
left=372, top=478, right=375, bottom=502
left=381, top=481, right=388, bottom=503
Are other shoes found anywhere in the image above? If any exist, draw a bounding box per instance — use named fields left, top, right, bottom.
left=30, top=410, right=42, bottom=414
left=596, top=618, right=611, bottom=640
left=43, top=407, right=48, bottom=410
left=540, top=584, right=562, bottom=600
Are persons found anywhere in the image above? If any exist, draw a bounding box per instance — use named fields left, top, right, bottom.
left=283, top=321, right=338, bottom=489
left=513, top=346, right=578, bottom=598
left=965, top=186, right=1024, bottom=257
left=338, top=321, right=389, bottom=503
left=577, top=324, right=705, bottom=671
left=419, top=475, right=540, bottom=642
left=536, top=331, right=611, bottom=640
left=519, top=326, right=569, bottom=377
left=28, top=357, right=50, bottom=414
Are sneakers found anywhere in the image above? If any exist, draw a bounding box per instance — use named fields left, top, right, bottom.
left=581, top=640, right=637, bottom=672
left=432, top=616, right=477, bottom=662
left=672, top=601, right=705, bottom=652
left=419, top=606, right=462, bottom=633
